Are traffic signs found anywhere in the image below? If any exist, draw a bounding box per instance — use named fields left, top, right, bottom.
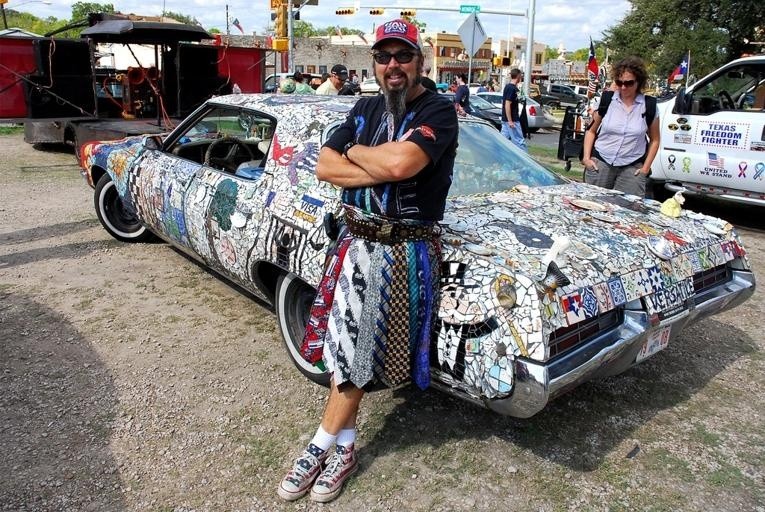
left=460, top=5, right=481, bottom=15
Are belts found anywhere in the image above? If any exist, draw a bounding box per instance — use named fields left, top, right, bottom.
left=343, top=209, right=436, bottom=243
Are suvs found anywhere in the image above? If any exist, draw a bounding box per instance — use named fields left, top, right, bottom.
left=558, top=53, right=765, bottom=207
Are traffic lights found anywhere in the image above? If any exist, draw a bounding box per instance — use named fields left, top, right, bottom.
left=336, top=8, right=355, bottom=14
left=492, top=57, right=511, bottom=68
left=370, top=9, right=384, bottom=15
left=401, top=9, right=416, bottom=16
left=270, top=5, right=288, bottom=39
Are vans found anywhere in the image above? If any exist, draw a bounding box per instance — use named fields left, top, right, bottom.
left=265, top=73, right=323, bottom=94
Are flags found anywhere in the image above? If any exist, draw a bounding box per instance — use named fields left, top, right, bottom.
left=588, top=38, right=599, bottom=93
left=668, top=52, right=689, bottom=84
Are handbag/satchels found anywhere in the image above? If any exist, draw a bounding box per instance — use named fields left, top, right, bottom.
left=461, top=94, right=467, bottom=103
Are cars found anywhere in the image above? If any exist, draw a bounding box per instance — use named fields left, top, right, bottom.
left=80, top=94, right=755, bottom=418
left=338, top=79, right=381, bottom=95
left=440, top=84, right=588, bottom=132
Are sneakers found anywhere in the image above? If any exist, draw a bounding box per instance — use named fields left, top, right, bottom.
left=311, top=446, right=359, bottom=503
left=278, top=444, right=329, bottom=501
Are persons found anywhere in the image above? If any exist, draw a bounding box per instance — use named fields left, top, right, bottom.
left=419, top=64, right=437, bottom=93
left=581, top=55, right=660, bottom=200
left=276, top=18, right=459, bottom=503
left=476, top=78, right=501, bottom=93
left=675, top=79, right=686, bottom=95
left=280, top=63, right=368, bottom=95
left=454, top=71, right=469, bottom=116
left=499, top=69, right=528, bottom=153
left=448, top=80, right=459, bottom=94
left=652, top=78, right=660, bottom=97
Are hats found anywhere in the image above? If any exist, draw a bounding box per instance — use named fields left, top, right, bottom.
left=331, top=65, right=349, bottom=80
left=371, top=19, right=423, bottom=49
left=509, top=68, right=520, bottom=75
left=280, top=79, right=296, bottom=93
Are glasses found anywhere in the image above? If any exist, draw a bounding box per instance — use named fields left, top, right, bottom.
left=373, top=51, right=418, bottom=63
left=614, top=79, right=636, bottom=87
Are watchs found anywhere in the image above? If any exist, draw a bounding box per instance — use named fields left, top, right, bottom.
left=342, top=141, right=358, bottom=157
